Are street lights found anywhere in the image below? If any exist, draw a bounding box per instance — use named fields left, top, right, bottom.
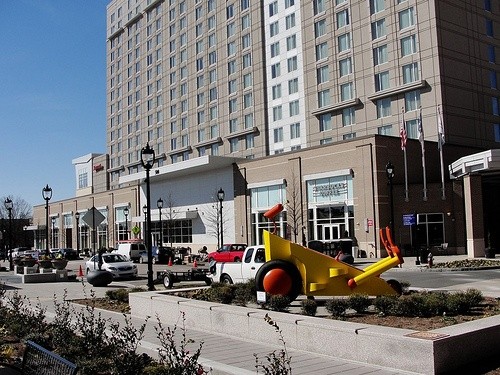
left=385, top=161, right=396, bottom=243
left=157, top=198, right=163, bottom=248
left=41, top=184, right=53, bottom=255
left=4, top=198, right=14, bottom=271
left=217, top=188, right=224, bottom=247
left=143, top=205, right=148, bottom=247
left=237, top=167, right=248, bottom=245
left=52, top=217, right=56, bottom=248
left=75, top=212, right=80, bottom=259
left=289, top=156, right=307, bottom=247
left=124, top=207, right=129, bottom=239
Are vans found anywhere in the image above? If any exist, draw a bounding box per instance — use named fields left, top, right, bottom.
left=111, top=243, right=146, bottom=262
left=140, top=142, right=156, bottom=291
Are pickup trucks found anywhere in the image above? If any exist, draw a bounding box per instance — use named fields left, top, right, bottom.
left=207, top=245, right=266, bottom=286
left=206, top=244, right=247, bottom=263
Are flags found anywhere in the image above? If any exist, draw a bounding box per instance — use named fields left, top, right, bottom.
left=400, top=109, right=407, bottom=150
left=418, top=108, right=425, bottom=145
left=437, top=107, right=445, bottom=149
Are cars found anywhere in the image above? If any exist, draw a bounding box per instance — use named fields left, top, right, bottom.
left=7, top=247, right=77, bottom=260
left=139, top=246, right=173, bottom=265
left=85, top=252, right=138, bottom=280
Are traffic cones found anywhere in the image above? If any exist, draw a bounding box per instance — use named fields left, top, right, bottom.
left=193, top=257, right=197, bottom=268
left=167, top=256, right=173, bottom=266
left=78, top=265, right=84, bottom=276
